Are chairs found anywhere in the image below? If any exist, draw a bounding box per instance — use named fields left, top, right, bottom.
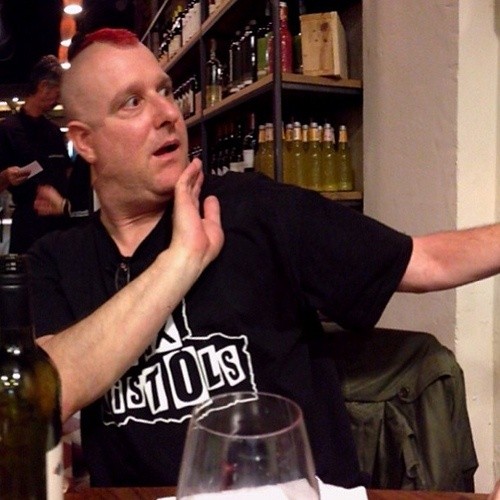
left=341, top=327, right=479, bottom=494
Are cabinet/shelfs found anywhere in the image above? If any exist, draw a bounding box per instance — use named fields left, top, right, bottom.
left=140, top=0, right=365, bottom=200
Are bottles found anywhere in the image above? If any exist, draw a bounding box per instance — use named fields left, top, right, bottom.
left=0, top=254, right=63, bottom=500
left=172, top=2, right=302, bottom=119
left=188, top=117, right=353, bottom=192
left=156, top=0, right=228, bottom=67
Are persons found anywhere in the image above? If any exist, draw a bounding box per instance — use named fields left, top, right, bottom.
left=0, top=18, right=500, bottom=486
left=1, top=55, right=101, bottom=254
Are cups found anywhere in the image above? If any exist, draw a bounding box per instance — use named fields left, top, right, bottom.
left=176, top=392, right=320, bottom=500
